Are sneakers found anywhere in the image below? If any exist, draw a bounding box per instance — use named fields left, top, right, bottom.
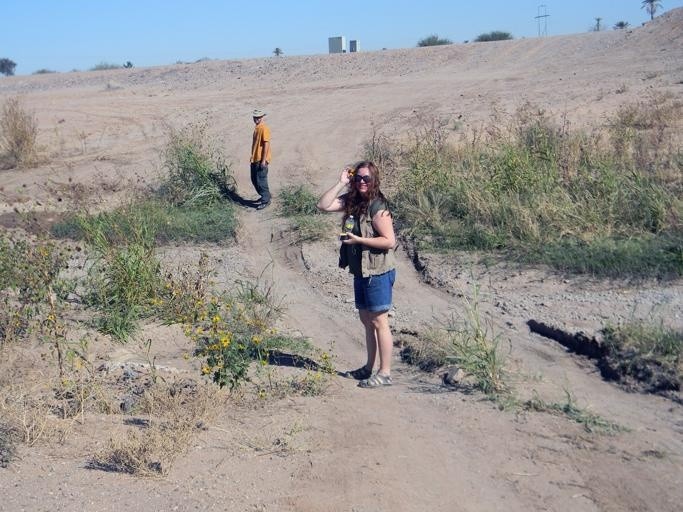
left=358, top=371, right=393, bottom=389
left=345, top=365, right=380, bottom=380
left=252, top=199, right=271, bottom=210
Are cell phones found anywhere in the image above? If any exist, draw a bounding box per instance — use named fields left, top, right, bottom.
left=338, top=233, right=353, bottom=240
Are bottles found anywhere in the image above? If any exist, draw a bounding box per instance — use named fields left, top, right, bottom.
left=342, top=214, right=354, bottom=233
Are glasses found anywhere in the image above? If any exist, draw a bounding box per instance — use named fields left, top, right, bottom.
left=354, top=175, right=370, bottom=183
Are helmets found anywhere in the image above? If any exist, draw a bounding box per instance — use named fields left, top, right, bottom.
left=252, top=109, right=266, bottom=118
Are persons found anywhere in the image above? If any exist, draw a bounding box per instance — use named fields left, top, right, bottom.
left=316, top=161, right=396, bottom=388
left=250, top=110, right=272, bottom=210
